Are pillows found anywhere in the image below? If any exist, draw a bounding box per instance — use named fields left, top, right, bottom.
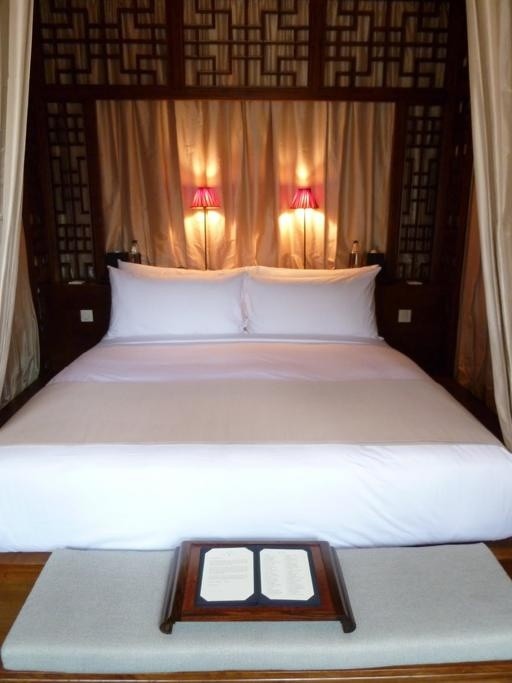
left=101, top=251, right=381, bottom=344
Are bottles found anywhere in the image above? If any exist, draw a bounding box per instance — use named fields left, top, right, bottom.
left=349, top=239, right=361, bottom=269
left=130, top=240, right=142, bottom=265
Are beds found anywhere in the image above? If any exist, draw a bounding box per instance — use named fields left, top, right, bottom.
left=1, top=254, right=512, bottom=552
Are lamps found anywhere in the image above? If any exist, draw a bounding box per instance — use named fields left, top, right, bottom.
left=192, top=186, right=222, bottom=271
left=289, top=187, right=319, bottom=270
